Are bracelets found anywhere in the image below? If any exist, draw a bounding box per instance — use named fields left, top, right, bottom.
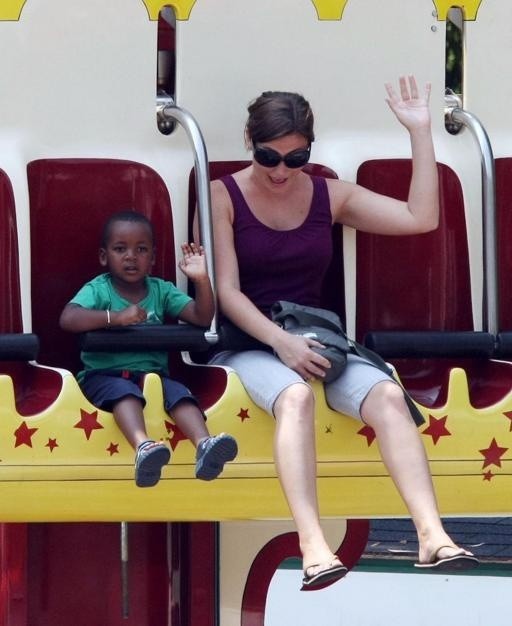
left=107, top=309, right=111, bottom=327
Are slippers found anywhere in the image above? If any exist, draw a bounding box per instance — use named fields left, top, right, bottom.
left=415, top=545, right=479, bottom=571
left=304, top=556, right=347, bottom=586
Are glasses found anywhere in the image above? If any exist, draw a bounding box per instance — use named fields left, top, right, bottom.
left=252, top=139, right=312, bottom=167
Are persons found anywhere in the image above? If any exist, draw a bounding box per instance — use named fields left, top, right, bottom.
left=192, top=74, right=484, bottom=591
left=58, top=210, right=239, bottom=488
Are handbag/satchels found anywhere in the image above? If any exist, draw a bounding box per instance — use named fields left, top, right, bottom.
left=273, top=301, right=349, bottom=382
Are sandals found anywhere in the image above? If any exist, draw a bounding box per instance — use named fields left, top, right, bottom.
left=195, top=435, right=237, bottom=480
left=134, top=440, right=170, bottom=487
